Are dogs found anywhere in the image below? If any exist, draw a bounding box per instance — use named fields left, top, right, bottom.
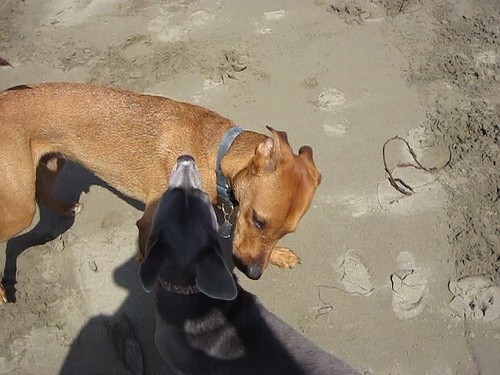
left=137, top=155, right=361, bottom=374
left=0, top=58, right=322, bottom=306
